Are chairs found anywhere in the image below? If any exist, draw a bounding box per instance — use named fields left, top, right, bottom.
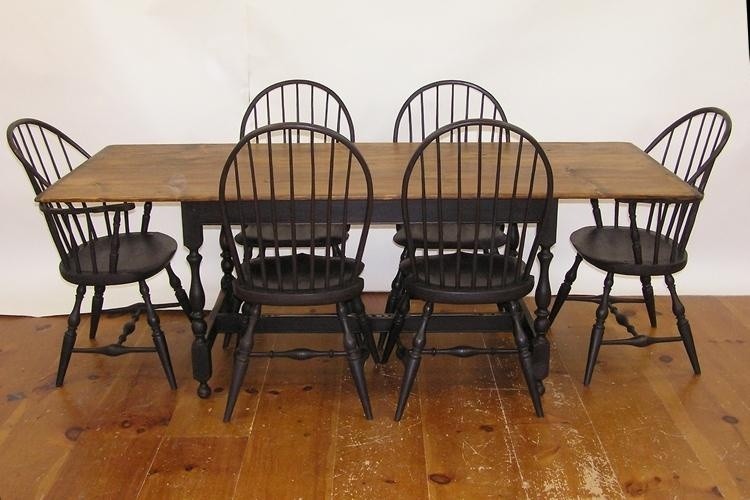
left=216, top=122, right=373, bottom=422
left=378, top=80, right=535, bottom=362
left=374, top=118, right=559, bottom=421
left=6, top=118, right=193, bottom=392
left=547, top=106, right=731, bottom=389
left=207, top=80, right=380, bottom=364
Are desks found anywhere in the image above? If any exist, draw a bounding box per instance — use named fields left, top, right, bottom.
left=35, top=141, right=702, bottom=398
